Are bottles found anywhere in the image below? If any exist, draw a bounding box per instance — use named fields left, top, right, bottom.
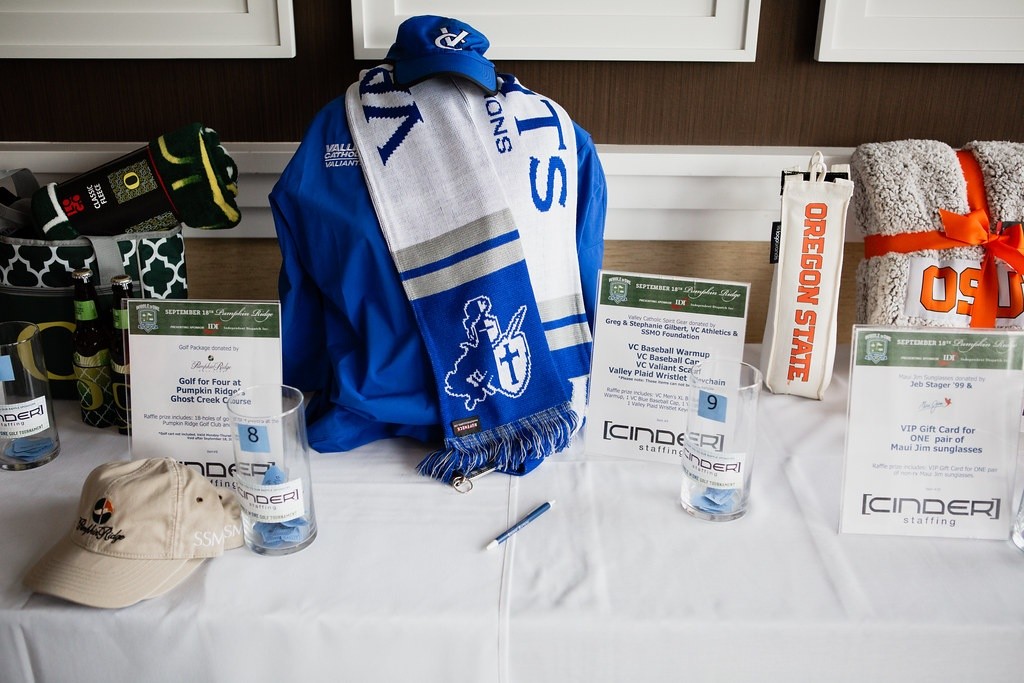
left=109, top=275, right=135, bottom=436
left=71, top=269, right=115, bottom=428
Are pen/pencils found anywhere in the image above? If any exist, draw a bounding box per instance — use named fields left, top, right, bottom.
left=486, top=498, right=556, bottom=552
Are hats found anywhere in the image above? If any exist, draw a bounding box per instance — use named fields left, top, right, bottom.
left=22, top=454, right=244, bottom=610
left=383, top=15, right=498, bottom=95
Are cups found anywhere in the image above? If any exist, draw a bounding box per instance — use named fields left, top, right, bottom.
left=679, top=360, right=763, bottom=521
left=1012, top=494, right=1024, bottom=551
left=228, top=384, right=317, bottom=555
left=0, top=322, right=60, bottom=471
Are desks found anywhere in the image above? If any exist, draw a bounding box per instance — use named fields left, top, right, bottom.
left=0, top=345, right=1022, bottom=682
